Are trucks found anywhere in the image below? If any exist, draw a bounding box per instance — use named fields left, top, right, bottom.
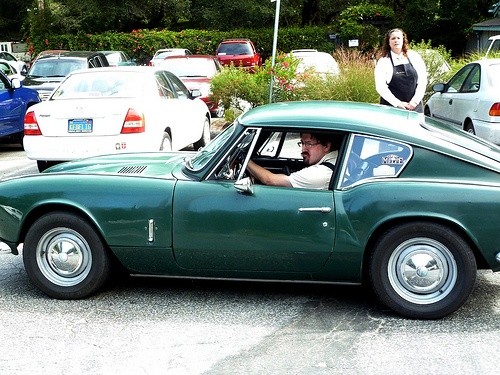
left=0, top=42, right=26, bottom=59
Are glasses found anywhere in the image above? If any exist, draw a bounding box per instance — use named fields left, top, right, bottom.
left=298, top=142, right=322, bottom=149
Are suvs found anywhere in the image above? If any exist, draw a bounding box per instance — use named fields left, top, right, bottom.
left=19, top=51, right=109, bottom=102
left=216, top=38, right=265, bottom=75
left=97, top=50, right=137, bottom=66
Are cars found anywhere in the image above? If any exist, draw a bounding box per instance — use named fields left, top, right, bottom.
left=0, top=69, right=43, bottom=146
left=23, top=66, right=212, bottom=174
left=275, top=48, right=341, bottom=93
left=416, top=48, right=453, bottom=86
left=0, top=100, right=500, bottom=320
left=30, top=50, right=68, bottom=67
left=0, top=50, right=28, bottom=88
left=480, top=35, right=500, bottom=61
left=159, top=55, right=227, bottom=118
left=424, top=58, right=500, bottom=147
left=148, top=48, right=193, bottom=67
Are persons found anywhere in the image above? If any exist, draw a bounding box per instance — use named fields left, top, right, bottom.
left=374, top=28, right=427, bottom=153
left=231, top=132, right=350, bottom=189
